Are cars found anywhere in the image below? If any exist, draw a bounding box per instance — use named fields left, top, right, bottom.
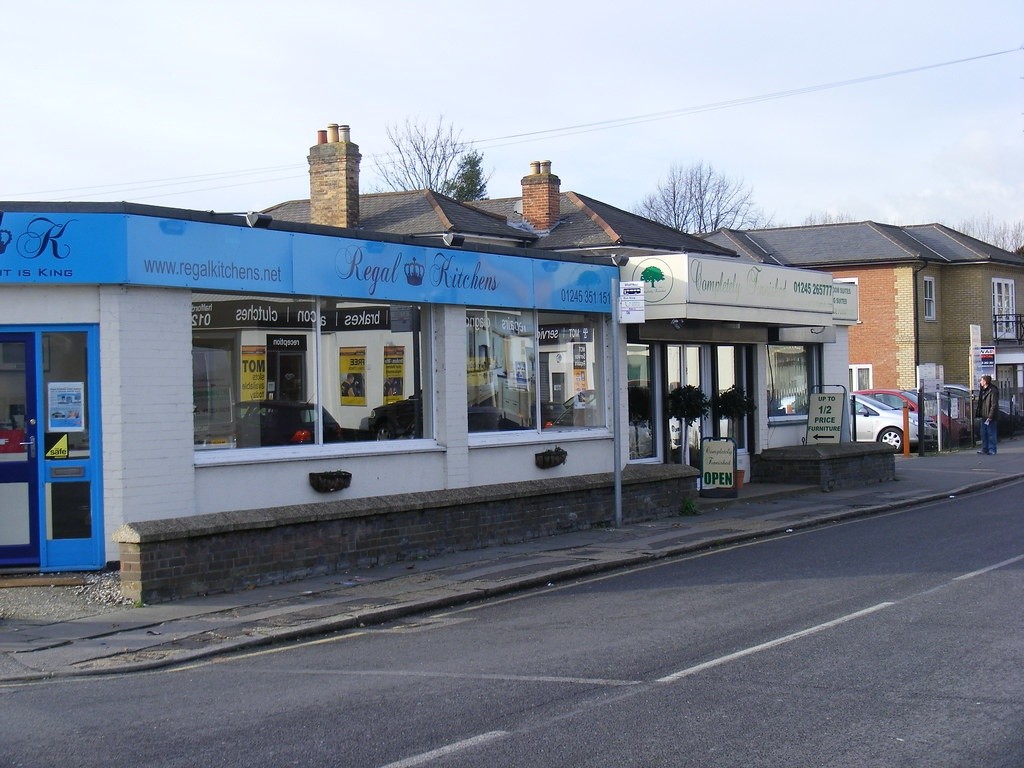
left=854, top=389, right=964, bottom=444
left=910, top=383, right=1020, bottom=441
left=849, top=393, right=942, bottom=454
left=367, top=390, right=424, bottom=442
left=229, top=400, right=341, bottom=447
left=525, top=389, right=600, bottom=431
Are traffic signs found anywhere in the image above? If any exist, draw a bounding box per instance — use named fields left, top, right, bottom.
left=804, top=384, right=852, bottom=445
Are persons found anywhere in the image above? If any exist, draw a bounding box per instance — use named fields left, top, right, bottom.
left=341, top=374, right=355, bottom=397
left=975, top=375, right=999, bottom=455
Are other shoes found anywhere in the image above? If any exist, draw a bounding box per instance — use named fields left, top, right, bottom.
left=976, top=450, right=985, bottom=454
left=987, top=451, right=995, bottom=455
